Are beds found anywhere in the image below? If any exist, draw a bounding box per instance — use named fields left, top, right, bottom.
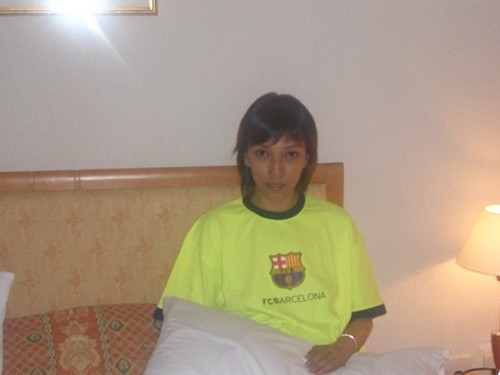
left=0, top=161, right=345, bottom=375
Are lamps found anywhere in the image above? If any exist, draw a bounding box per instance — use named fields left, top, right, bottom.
left=456, top=203, right=500, bottom=369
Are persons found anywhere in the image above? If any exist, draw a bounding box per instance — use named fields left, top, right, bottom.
left=151, top=92, right=387, bottom=375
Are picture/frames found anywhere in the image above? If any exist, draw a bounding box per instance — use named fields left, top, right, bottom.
left=0, top=0, right=158, bottom=16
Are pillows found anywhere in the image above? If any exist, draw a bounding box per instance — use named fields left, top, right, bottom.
left=3, top=302, right=163, bottom=375
left=141, top=295, right=451, bottom=375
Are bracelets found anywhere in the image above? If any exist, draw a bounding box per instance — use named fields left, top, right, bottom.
left=341, top=334, right=358, bottom=352
